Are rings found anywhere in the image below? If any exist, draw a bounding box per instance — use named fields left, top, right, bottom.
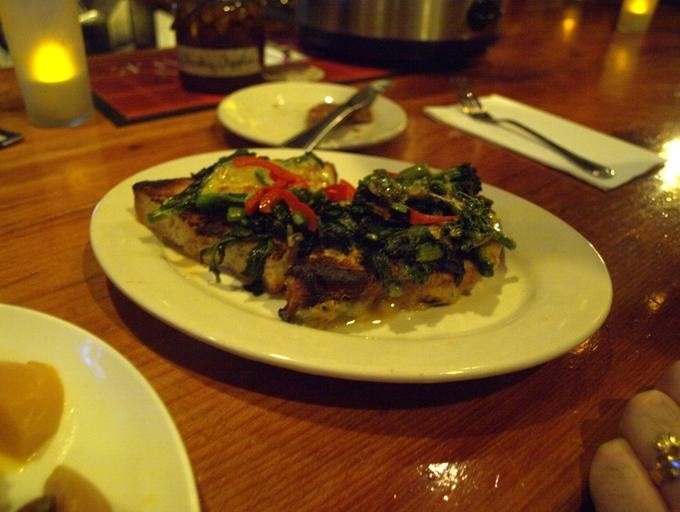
left=649, top=432, right=678, bottom=488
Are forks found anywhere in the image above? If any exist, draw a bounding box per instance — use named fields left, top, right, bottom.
left=450, top=77, right=616, bottom=180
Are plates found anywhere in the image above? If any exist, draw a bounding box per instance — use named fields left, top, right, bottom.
left=1, top=301, right=202, bottom=512
left=88, top=145, right=616, bottom=386
left=214, top=79, right=409, bottom=150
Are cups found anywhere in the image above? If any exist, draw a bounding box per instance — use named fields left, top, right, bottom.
left=1, top=0, right=95, bottom=132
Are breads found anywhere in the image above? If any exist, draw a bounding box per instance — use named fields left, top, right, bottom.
left=131, top=161, right=502, bottom=323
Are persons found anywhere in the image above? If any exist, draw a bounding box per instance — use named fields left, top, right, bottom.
left=588, top=362, right=680, bottom=511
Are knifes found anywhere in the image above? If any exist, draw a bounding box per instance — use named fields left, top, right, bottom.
left=280, top=84, right=379, bottom=150
